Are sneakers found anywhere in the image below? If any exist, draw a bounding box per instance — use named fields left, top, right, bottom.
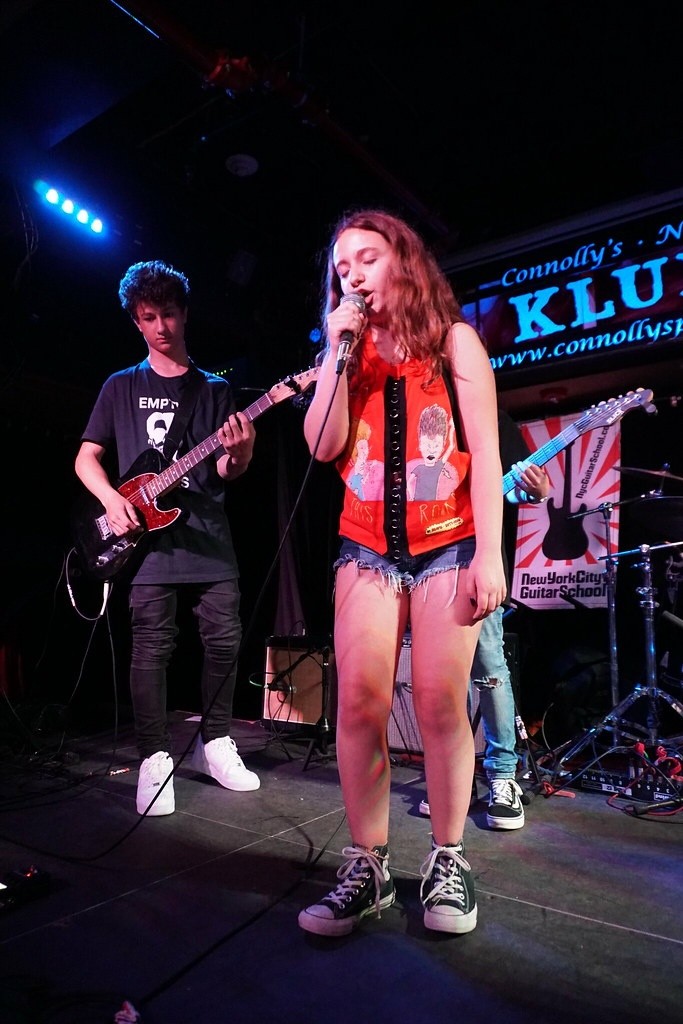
left=136, top=751, right=175, bottom=816
left=298, top=843, right=396, bottom=936
left=487, top=778, right=524, bottom=829
left=419, top=835, right=477, bottom=934
left=419, top=774, right=478, bottom=816
left=190, top=731, right=260, bottom=792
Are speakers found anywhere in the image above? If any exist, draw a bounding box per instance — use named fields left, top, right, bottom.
left=262, top=638, right=337, bottom=730
left=386, top=633, right=488, bottom=758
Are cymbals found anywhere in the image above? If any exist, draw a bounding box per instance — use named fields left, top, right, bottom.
left=610, top=462, right=683, bottom=484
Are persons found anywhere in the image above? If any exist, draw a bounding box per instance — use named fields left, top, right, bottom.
left=419, top=420, right=550, bottom=829
left=299, top=210, right=506, bottom=935
left=74, top=260, right=260, bottom=816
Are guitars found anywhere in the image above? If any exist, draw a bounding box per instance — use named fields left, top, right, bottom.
left=65, top=352, right=334, bottom=587
left=502, top=384, right=656, bottom=496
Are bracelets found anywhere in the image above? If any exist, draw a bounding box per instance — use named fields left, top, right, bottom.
left=535, top=497, right=548, bottom=503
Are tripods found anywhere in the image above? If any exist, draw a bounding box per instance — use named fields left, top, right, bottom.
left=515, top=483, right=683, bottom=792
left=272, top=633, right=337, bottom=772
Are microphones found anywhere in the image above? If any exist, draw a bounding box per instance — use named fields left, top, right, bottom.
left=336, top=293, right=366, bottom=375
left=264, top=683, right=297, bottom=693
